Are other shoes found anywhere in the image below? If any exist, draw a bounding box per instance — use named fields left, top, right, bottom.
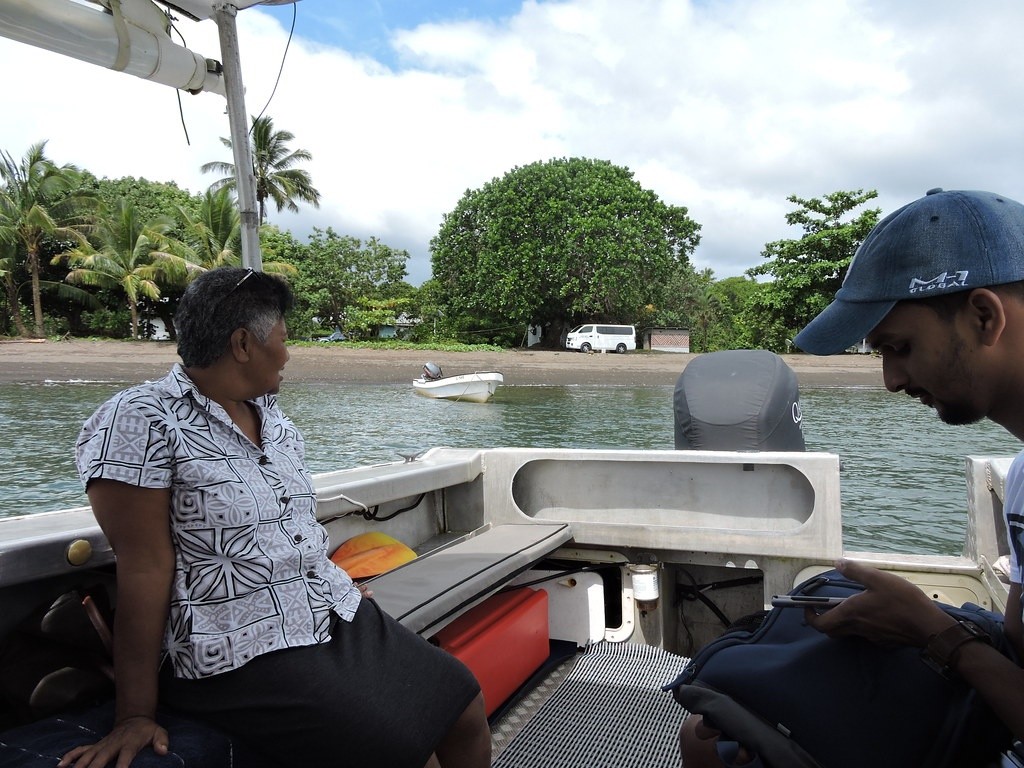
left=29, top=664, right=112, bottom=713
left=41, top=584, right=111, bottom=637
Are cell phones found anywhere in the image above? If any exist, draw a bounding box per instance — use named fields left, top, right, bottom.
left=771, top=595, right=848, bottom=607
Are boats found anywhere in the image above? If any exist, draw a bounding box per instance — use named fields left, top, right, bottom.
left=411, top=361, right=503, bottom=404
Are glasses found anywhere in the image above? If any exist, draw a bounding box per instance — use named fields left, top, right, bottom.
left=211, top=267, right=262, bottom=315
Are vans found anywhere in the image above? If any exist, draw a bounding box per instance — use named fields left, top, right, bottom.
left=566, top=323, right=638, bottom=355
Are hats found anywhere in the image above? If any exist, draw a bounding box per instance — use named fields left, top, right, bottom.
left=792, top=187, right=1024, bottom=356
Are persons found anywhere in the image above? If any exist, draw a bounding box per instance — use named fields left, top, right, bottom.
left=57, top=268, right=491, bottom=768
left=678, top=189, right=1024, bottom=768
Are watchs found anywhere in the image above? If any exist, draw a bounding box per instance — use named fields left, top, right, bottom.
left=917, top=619, right=992, bottom=678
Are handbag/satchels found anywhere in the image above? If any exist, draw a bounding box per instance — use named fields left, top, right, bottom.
left=662, top=568, right=1024, bottom=768
